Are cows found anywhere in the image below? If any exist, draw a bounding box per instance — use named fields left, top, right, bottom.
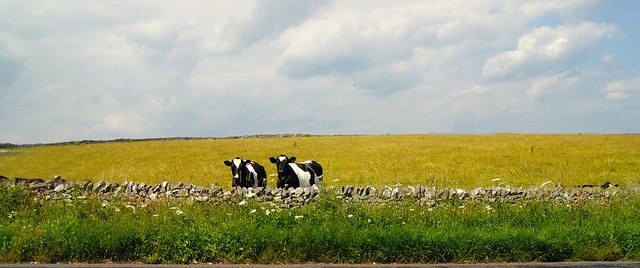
left=223, top=156, right=266, bottom=189
left=269, top=154, right=324, bottom=190
